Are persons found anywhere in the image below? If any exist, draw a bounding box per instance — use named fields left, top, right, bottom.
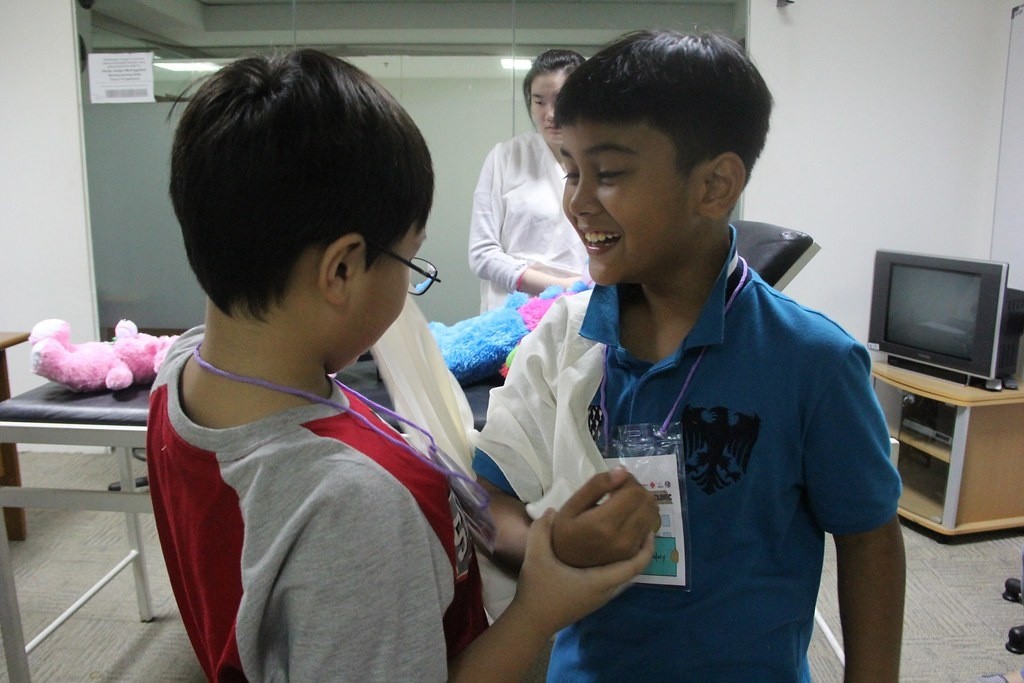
left=467, top=27, right=905, bottom=683
left=147, top=50, right=655, bottom=683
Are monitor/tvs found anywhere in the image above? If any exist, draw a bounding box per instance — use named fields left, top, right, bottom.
left=867, top=249, right=1024, bottom=386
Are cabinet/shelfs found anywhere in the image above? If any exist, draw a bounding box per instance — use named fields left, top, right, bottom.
left=868, top=359, right=1024, bottom=545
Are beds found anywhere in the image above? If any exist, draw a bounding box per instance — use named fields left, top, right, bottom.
left=0, top=220, right=821, bottom=682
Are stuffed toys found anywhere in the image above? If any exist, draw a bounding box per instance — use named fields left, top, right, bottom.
left=28, top=318, right=180, bottom=392
left=429, top=281, right=595, bottom=386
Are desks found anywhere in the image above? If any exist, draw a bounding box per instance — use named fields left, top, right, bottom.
left=0, top=332, right=34, bottom=541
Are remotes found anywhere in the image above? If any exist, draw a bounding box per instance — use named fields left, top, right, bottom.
left=1001, top=375, right=1018, bottom=390
left=985, top=379, right=1002, bottom=390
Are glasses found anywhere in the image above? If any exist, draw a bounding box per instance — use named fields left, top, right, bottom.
left=365, top=235, right=441, bottom=297
